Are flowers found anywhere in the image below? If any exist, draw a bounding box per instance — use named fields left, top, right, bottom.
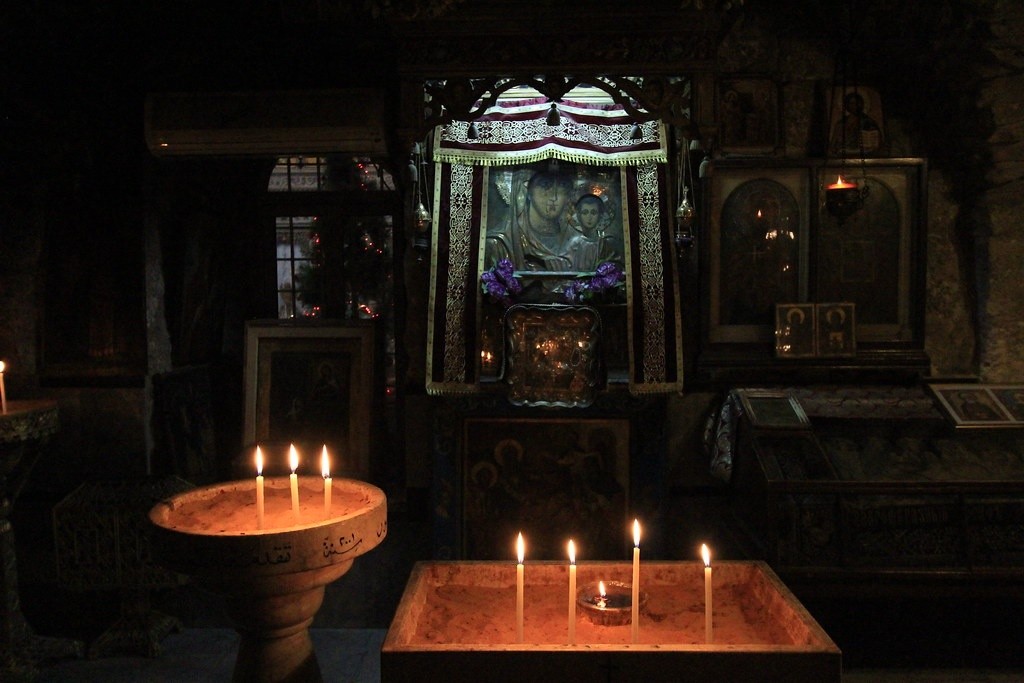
left=481, top=257, right=533, bottom=308
left=565, top=257, right=623, bottom=303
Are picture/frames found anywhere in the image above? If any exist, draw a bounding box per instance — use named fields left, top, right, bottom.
left=243, top=319, right=376, bottom=481
left=712, top=70, right=786, bottom=159
left=738, top=387, right=837, bottom=484
left=927, top=382, right=1024, bottom=430
left=703, top=159, right=927, bottom=359
left=433, top=395, right=670, bottom=561
left=821, top=79, right=892, bottom=159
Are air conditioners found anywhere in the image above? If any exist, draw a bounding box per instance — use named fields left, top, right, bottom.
left=143, top=82, right=388, bottom=160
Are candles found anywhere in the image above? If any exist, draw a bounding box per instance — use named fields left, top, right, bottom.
left=568, top=564, right=578, bottom=640
left=290, top=474, right=299, bottom=511
left=517, top=564, right=525, bottom=637
left=705, top=567, right=712, bottom=642
left=255, top=475, right=265, bottom=520
left=631, top=547, right=640, bottom=640
left=325, top=478, right=333, bottom=515
left=0, top=373, right=8, bottom=414
